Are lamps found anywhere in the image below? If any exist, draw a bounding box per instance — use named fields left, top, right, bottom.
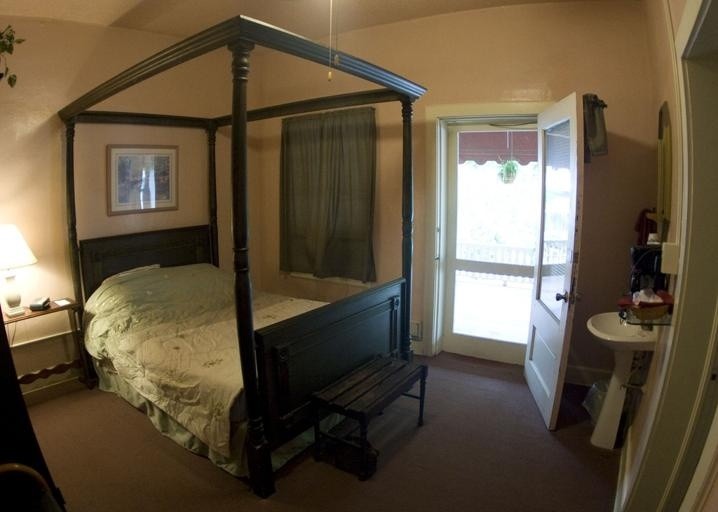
left=0, top=223, right=38, bottom=318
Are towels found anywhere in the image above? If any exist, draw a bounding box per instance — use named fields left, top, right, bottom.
left=635, top=208, right=657, bottom=246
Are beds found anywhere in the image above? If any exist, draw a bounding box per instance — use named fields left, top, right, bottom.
left=56, top=13, right=432, bottom=499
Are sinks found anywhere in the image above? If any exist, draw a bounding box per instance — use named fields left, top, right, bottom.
left=587, top=311, right=657, bottom=351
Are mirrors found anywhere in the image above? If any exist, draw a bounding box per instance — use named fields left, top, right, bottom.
left=658, top=101, right=673, bottom=245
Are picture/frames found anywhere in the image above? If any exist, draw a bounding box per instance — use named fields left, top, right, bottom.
left=105, top=144, right=180, bottom=217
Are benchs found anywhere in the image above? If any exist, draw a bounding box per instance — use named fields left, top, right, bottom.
left=311, top=355, right=428, bottom=473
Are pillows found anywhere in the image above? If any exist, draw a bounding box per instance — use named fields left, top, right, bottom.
left=99, top=261, right=235, bottom=328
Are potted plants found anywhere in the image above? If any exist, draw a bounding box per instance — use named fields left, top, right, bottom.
left=0, top=25, right=27, bottom=88
left=498, top=160, right=519, bottom=184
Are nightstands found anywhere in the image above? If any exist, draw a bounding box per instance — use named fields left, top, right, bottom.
left=2, top=297, right=98, bottom=390
